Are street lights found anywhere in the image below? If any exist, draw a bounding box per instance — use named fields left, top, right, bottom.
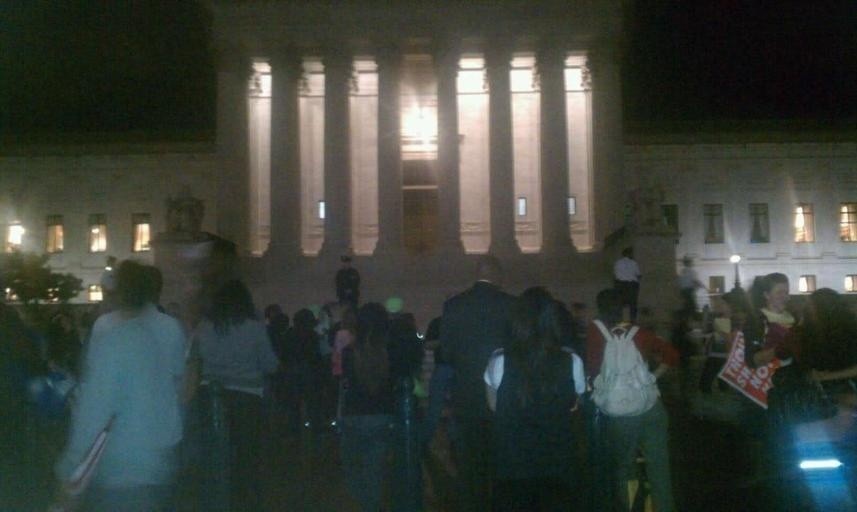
left=729, top=255, right=741, bottom=285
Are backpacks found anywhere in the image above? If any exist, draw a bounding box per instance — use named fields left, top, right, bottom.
left=589, top=319, right=660, bottom=418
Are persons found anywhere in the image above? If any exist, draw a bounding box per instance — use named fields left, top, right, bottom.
left=0, top=245, right=856, bottom=510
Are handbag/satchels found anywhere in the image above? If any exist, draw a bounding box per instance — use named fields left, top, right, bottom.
left=52, top=426, right=111, bottom=501
left=717, top=332, right=778, bottom=411
